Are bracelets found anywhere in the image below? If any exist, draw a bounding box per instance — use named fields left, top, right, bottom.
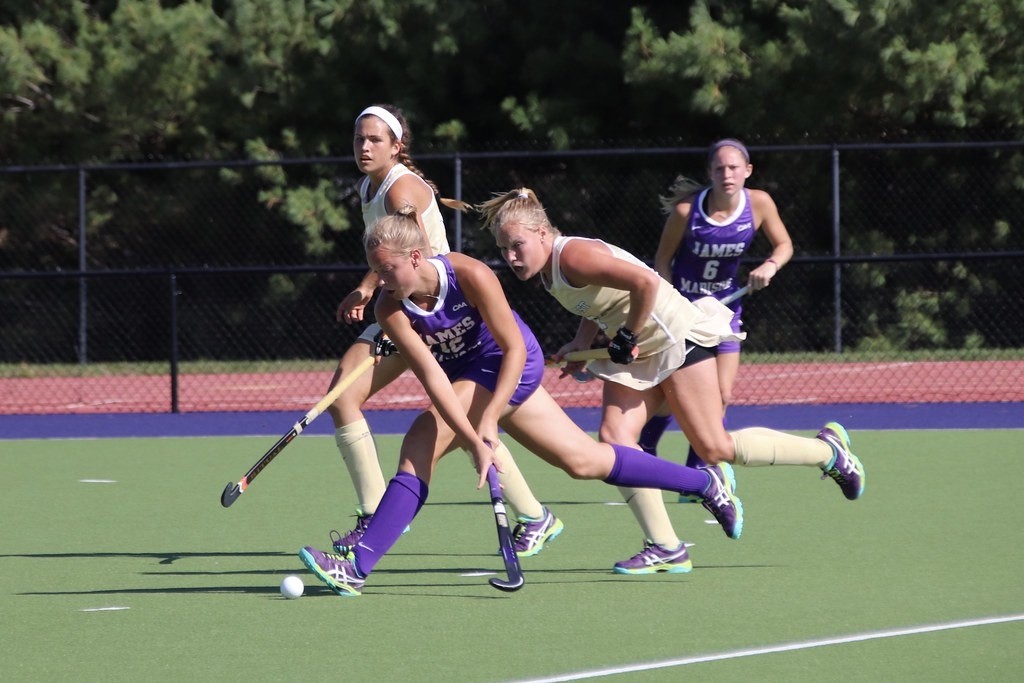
left=766, top=259, right=778, bottom=271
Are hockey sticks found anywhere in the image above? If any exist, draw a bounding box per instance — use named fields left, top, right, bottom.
left=541, top=344, right=641, bottom=365
left=484, top=441, right=524, bottom=592
left=719, top=286, right=749, bottom=305
left=220, top=349, right=375, bottom=508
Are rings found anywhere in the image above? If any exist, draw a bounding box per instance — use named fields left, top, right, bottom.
left=748, top=284, right=752, bottom=287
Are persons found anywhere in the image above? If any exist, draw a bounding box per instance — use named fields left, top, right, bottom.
left=299, top=205, right=745, bottom=596
left=327, top=105, right=564, bottom=558
left=639, top=140, right=795, bottom=503
left=481, top=188, right=866, bottom=575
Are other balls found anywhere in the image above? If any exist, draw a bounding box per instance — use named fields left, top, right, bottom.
left=280, top=576, right=305, bottom=599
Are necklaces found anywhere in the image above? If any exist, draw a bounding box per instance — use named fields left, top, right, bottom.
left=424, top=295, right=438, bottom=299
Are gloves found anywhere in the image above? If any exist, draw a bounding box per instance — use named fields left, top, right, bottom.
left=373, top=329, right=399, bottom=358
left=607, top=326, right=639, bottom=365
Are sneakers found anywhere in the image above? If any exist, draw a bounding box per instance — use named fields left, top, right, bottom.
left=614, top=539, right=693, bottom=575
left=299, top=531, right=366, bottom=597
left=678, top=491, right=702, bottom=503
left=697, top=462, right=744, bottom=539
left=498, top=506, right=564, bottom=557
left=817, top=422, right=864, bottom=501
left=332, top=506, right=411, bottom=553
